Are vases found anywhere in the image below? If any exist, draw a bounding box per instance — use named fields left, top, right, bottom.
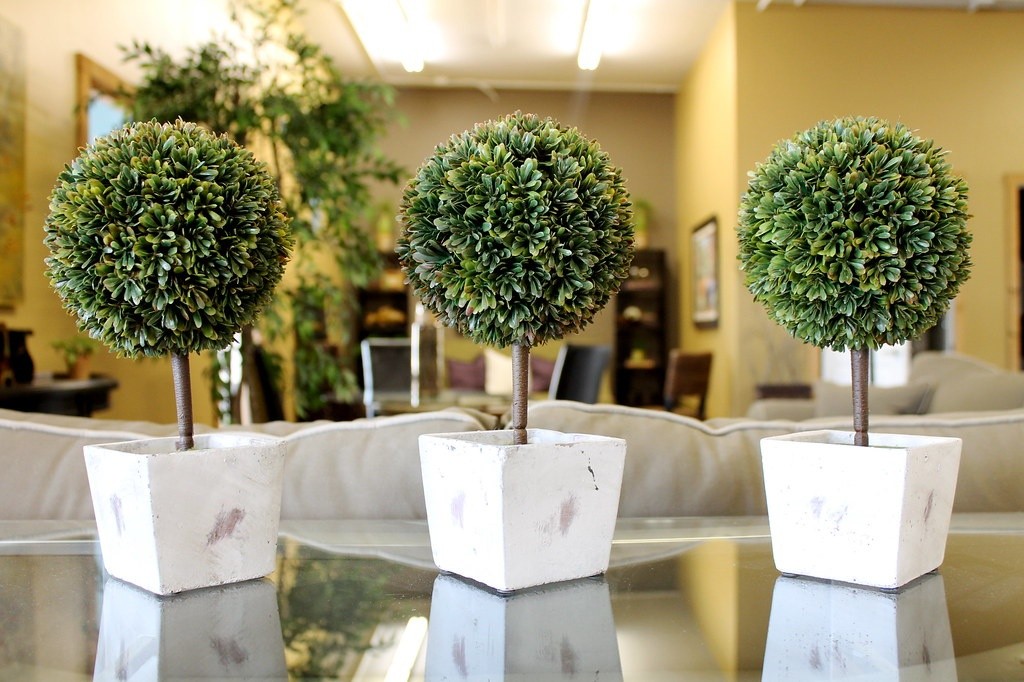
left=95, top=569, right=290, bottom=682
left=760, top=573, right=959, bottom=682
left=427, top=572, right=625, bottom=682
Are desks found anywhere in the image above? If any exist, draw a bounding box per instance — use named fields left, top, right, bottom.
left=0, top=538, right=1023, bottom=682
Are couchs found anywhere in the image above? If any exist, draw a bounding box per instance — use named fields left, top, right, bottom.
left=1, top=349, right=1024, bottom=538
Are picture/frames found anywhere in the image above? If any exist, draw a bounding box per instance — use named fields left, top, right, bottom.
left=75, top=50, right=138, bottom=158
left=690, top=217, right=720, bottom=326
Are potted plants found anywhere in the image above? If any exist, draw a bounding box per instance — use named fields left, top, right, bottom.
left=42, top=114, right=297, bottom=597
left=50, top=335, right=95, bottom=381
left=394, top=108, right=635, bottom=593
left=732, top=112, right=976, bottom=588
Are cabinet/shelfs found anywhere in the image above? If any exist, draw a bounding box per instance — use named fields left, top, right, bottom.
left=0, top=321, right=119, bottom=415
left=611, top=248, right=670, bottom=408
left=354, top=250, right=418, bottom=387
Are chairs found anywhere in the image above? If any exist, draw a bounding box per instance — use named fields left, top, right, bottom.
left=664, top=350, right=712, bottom=421
left=361, top=337, right=411, bottom=417
left=549, top=344, right=612, bottom=404
left=240, top=344, right=284, bottom=424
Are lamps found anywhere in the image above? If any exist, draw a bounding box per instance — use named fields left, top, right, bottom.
left=577, top=1, right=609, bottom=71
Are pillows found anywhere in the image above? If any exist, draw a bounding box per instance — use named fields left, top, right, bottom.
left=811, top=377, right=883, bottom=417
left=447, top=348, right=554, bottom=398
